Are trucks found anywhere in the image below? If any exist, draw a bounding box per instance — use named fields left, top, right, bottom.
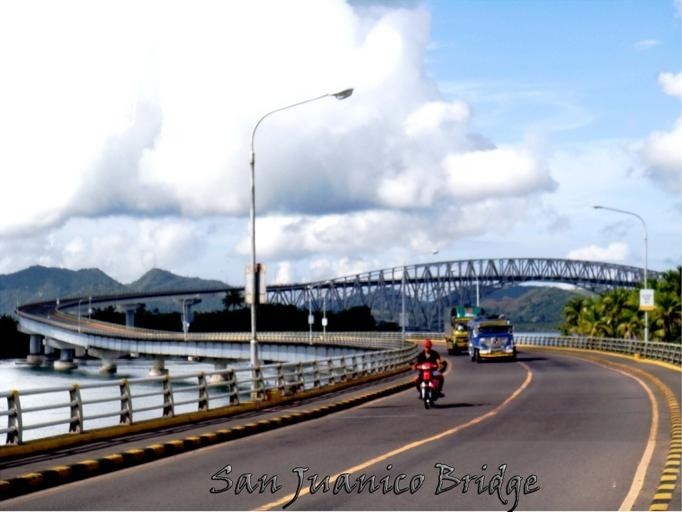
left=444, top=306, right=484, bottom=357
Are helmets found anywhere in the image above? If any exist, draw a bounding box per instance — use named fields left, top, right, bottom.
left=424, top=340, right=432, bottom=347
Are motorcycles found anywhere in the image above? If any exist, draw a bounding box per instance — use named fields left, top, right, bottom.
left=412, top=363, right=444, bottom=409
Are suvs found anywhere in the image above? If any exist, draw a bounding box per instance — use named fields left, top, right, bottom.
left=466, top=317, right=517, bottom=361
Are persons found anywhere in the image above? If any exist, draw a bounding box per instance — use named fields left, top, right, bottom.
left=416, top=339, right=444, bottom=398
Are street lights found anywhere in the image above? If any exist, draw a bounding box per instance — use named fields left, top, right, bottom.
left=594, top=202, right=650, bottom=352
left=248, top=88, right=355, bottom=396
